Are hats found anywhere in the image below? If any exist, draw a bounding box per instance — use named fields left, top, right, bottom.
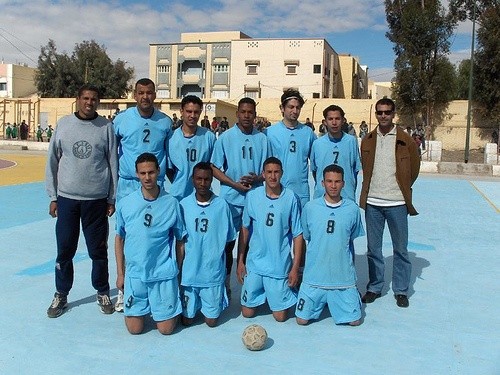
left=37, top=124, right=40, bottom=126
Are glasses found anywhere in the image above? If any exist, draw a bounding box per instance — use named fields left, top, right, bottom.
left=376, top=110, right=392, bottom=115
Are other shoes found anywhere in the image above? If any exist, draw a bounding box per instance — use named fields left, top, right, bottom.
left=225, top=284, right=231, bottom=296
left=296, top=275, right=302, bottom=290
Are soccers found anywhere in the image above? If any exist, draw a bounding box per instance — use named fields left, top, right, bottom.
left=241, top=323, right=268, bottom=351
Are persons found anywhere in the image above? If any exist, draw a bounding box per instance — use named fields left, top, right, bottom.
left=5, top=120, right=54, bottom=142
left=103, top=78, right=368, bottom=335
left=402, top=124, right=426, bottom=160
left=359, top=98, right=422, bottom=307
left=45, top=85, right=118, bottom=318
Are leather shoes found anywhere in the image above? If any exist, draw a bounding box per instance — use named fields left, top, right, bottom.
left=394, top=294, right=409, bottom=307
left=361, top=291, right=381, bottom=303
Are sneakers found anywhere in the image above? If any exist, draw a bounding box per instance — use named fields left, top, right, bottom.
left=47, top=291, right=68, bottom=318
left=97, top=292, right=115, bottom=314
left=115, top=290, right=125, bottom=312
